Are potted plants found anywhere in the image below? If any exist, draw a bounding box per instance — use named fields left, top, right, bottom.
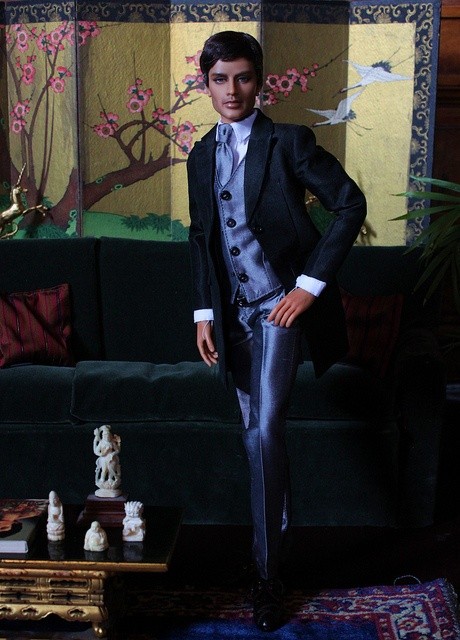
left=389, top=175, right=459, bottom=519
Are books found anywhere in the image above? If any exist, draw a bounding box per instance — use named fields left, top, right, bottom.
left=1, top=498, right=50, bottom=554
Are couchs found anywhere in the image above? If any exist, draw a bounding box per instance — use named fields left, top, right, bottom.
left=2, top=237, right=446, bottom=561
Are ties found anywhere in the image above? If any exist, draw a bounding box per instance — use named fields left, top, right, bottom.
left=214, top=123, right=234, bottom=186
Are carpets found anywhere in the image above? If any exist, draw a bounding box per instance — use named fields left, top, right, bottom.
left=0, top=579, right=459, bottom=634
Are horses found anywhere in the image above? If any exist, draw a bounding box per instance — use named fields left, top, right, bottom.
left=0, top=184, right=52, bottom=236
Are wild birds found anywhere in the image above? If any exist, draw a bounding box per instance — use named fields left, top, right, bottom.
left=304, top=45, right=414, bottom=138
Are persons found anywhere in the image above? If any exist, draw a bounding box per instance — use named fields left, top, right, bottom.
left=186, top=31, right=367, bottom=631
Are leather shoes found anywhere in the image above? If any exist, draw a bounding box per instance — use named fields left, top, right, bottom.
left=252, top=589, right=284, bottom=631
